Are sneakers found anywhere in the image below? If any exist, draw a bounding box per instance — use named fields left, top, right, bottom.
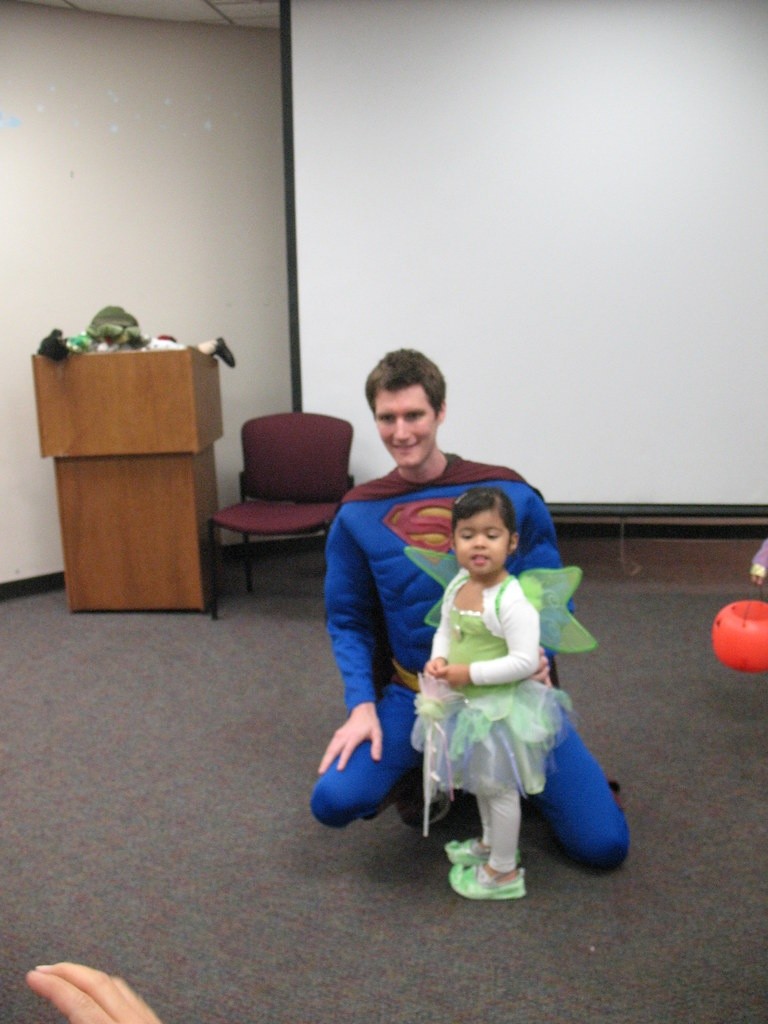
left=442, top=838, right=520, bottom=867
left=447, top=863, right=525, bottom=899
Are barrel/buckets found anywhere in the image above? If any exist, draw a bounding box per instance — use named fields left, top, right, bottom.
left=712, top=580, right=767, bottom=676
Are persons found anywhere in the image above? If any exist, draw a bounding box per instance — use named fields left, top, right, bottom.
left=310, top=348, right=629, bottom=878
left=413, top=488, right=541, bottom=900
left=25, top=960, right=164, bottom=1024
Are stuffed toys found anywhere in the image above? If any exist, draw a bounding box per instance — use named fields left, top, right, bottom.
left=36, top=305, right=235, bottom=369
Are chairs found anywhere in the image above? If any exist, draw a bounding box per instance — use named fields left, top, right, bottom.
left=207, top=410, right=356, bottom=620
